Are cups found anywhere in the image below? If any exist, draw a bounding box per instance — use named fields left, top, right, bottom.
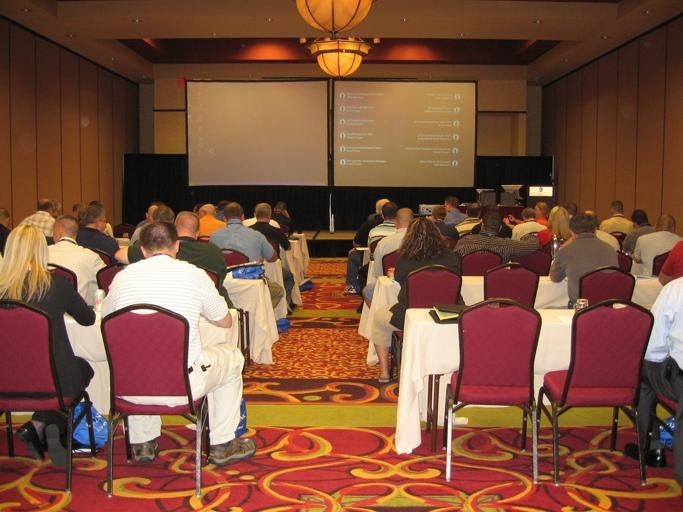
left=123, top=233, right=128, bottom=237
left=573, top=299, right=588, bottom=310
left=93, top=289, right=105, bottom=312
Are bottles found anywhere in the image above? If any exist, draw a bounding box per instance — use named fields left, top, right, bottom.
left=551, top=235, right=559, bottom=258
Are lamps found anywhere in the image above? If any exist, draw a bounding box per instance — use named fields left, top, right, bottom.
left=295, top=0, right=373, bottom=32
left=307, top=32, right=372, bottom=80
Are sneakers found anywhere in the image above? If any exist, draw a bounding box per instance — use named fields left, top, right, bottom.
left=344, top=286, right=356, bottom=293
left=133, top=440, right=158, bottom=463
left=208, top=438, right=255, bottom=464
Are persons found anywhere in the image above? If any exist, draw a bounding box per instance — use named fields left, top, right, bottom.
left=0, top=195, right=313, bottom=468
left=341, top=194, right=683, bottom=467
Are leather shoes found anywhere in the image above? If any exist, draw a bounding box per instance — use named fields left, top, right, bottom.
left=17, top=421, right=45, bottom=461
left=625, top=443, right=666, bottom=467
left=46, top=424, right=67, bottom=465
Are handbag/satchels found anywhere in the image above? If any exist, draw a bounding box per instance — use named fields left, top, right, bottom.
left=232, top=265, right=264, bottom=279
left=235, top=400, right=246, bottom=437
left=73, top=401, right=108, bottom=449
